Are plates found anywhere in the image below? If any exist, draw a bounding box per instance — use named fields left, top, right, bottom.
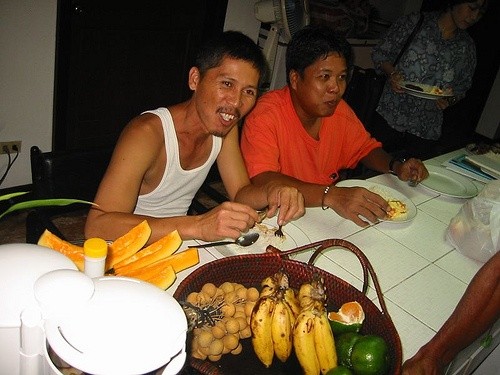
left=335, top=179, right=417, bottom=223
left=396, top=80, right=455, bottom=100
left=415, top=165, right=478, bottom=198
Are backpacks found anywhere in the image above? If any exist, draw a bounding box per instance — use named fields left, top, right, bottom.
left=310, top=1, right=369, bottom=37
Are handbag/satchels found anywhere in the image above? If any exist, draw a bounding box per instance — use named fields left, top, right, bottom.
left=443, top=179, right=500, bottom=264
left=355, top=79, right=387, bottom=128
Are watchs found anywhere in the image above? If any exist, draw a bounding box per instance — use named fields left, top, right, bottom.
left=389, top=158, right=405, bottom=176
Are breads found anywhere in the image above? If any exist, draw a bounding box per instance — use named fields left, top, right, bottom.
left=255, top=208, right=286, bottom=240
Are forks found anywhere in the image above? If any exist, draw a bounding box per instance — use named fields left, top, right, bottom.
left=275, top=225, right=284, bottom=239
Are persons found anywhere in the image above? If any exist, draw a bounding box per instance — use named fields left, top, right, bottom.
left=84, top=31, right=304, bottom=247
left=241, top=24, right=428, bottom=228
left=371, top=0, right=488, bottom=162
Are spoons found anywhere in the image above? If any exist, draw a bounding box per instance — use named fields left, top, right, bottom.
left=187, top=232, right=259, bottom=249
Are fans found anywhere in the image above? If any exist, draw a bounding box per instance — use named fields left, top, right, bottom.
left=254, top=0, right=310, bottom=96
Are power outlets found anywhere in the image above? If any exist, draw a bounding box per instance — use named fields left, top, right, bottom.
left=0, top=141, right=22, bottom=154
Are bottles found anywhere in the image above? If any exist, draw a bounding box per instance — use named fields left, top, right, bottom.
left=83, top=238, right=108, bottom=278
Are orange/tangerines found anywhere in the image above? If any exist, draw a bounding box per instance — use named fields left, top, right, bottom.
left=327, top=301, right=392, bottom=375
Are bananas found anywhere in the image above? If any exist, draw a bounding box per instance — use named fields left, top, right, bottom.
left=294, top=278, right=337, bottom=375
left=251, top=271, right=300, bottom=367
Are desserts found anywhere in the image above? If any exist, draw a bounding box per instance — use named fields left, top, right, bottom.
left=430, top=79, right=453, bottom=94
left=386, top=201, right=406, bottom=217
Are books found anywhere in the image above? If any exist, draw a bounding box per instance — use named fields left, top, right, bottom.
left=441, top=150, right=500, bottom=183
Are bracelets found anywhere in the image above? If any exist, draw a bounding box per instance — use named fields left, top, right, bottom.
left=446, top=96, right=456, bottom=106
left=390, top=70, right=401, bottom=78
left=322, top=184, right=333, bottom=211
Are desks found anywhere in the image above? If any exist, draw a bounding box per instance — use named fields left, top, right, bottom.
left=163, top=147, right=486, bottom=367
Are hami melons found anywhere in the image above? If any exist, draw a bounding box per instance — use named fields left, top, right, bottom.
left=38, top=220, right=200, bottom=291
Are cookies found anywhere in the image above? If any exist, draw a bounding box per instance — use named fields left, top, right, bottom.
left=405, top=84, right=424, bottom=92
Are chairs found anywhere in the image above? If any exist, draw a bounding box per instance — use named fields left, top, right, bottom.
left=26, top=146, right=230, bottom=248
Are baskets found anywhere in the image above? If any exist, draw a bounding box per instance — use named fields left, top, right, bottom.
left=173, top=239, right=403, bottom=375
